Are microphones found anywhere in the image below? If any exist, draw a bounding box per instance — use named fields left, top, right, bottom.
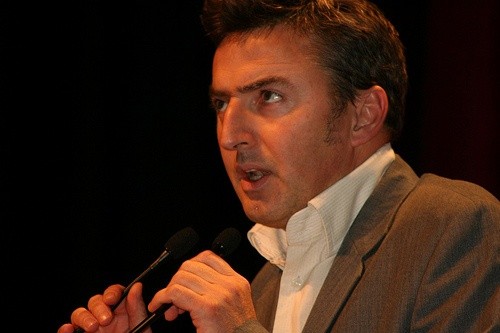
left=127, top=228, right=242, bottom=333
left=75, top=227, right=198, bottom=333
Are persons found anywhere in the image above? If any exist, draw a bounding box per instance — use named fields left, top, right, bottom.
left=56, top=0, right=500, bottom=333
